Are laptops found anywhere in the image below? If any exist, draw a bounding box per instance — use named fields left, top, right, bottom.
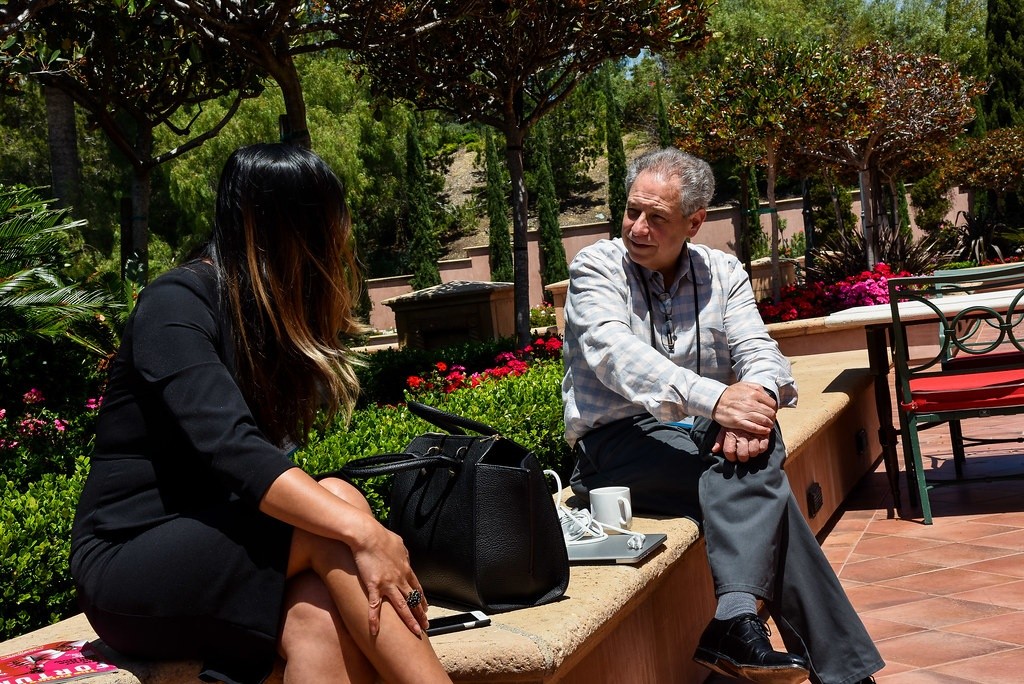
left=566, top=533, right=667, bottom=565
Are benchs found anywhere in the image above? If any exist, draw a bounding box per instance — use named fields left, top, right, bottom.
left=888, top=260, right=1024, bottom=525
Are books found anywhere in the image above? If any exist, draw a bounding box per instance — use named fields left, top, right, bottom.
left=0, top=639, right=118, bottom=684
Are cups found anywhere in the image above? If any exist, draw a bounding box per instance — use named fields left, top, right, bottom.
left=589, top=486, right=632, bottom=534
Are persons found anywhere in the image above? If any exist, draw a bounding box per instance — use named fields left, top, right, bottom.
left=561, top=146, right=886, bottom=684
left=68, top=143, right=453, bottom=684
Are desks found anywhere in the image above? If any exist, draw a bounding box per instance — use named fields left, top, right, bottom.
left=823, top=288, right=1024, bottom=523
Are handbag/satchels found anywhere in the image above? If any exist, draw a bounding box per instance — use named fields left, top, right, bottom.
left=340, top=401, right=570, bottom=615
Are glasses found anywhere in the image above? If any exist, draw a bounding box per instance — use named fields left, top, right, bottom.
left=652, top=290, right=677, bottom=350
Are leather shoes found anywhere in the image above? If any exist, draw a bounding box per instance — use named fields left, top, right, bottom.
left=693, top=612, right=810, bottom=684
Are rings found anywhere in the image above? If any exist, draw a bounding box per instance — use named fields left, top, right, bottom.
left=406, top=589, right=423, bottom=608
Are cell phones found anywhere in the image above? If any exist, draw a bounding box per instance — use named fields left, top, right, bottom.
left=425, top=610, right=491, bottom=636
